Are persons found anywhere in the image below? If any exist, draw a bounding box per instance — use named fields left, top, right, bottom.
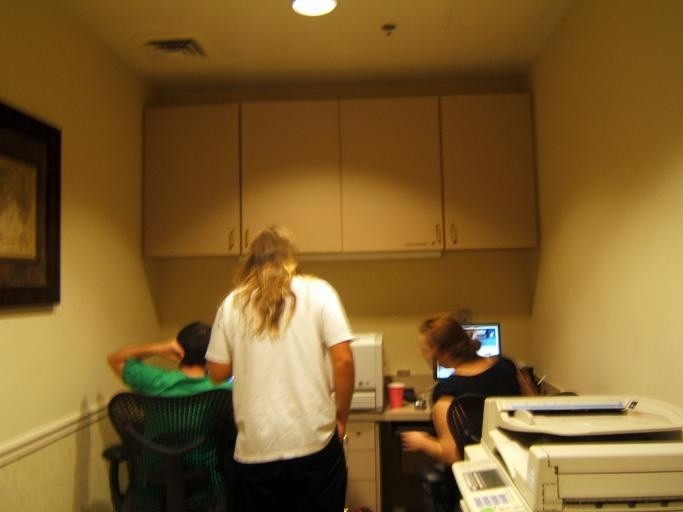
left=203, top=226, right=356, bottom=512
left=400, top=314, right=538, bottom=512
left=107, top=322, right=233, bottom=512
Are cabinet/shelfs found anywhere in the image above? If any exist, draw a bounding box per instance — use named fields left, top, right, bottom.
left=334, top=416, right=384, bottom=511
left=141, top=91, right=342, bottom=259
left=337, top=90, right=540, bottom=255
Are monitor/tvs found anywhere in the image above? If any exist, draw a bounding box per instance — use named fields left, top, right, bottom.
left=434, top=322, right=501, bottom=379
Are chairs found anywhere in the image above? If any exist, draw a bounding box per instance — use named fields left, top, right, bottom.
left=411, top=391, right=488, bottom=511
left=97, top=384, right=237, bottom=511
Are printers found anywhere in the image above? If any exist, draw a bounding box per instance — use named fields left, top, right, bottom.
left=451, top=396, right=682, bottom=512
left=333, top=333, right=384, bottom=411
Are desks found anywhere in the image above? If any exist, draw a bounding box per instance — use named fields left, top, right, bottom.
left=382, top=392, right=433, bottom=421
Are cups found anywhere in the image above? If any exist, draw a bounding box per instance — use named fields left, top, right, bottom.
left=387, top=382, right=405, bottom=408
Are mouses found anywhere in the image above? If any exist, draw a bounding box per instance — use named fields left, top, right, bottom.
left=415, top=399, right=426, bottom=409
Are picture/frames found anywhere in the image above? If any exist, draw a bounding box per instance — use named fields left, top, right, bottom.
left=0, top=98, right=64, bottom=305
